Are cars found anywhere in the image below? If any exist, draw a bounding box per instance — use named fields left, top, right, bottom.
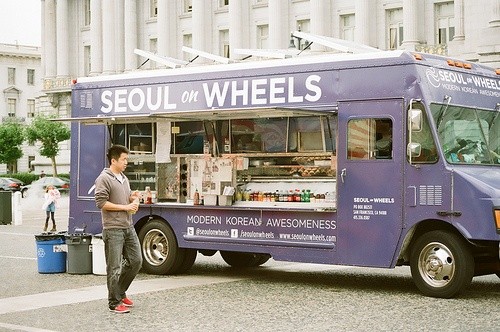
left=19, top=177, right=69, bottom=199
left=0, top=178, right=25, bottom=193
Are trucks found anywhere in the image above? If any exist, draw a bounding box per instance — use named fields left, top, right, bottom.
left=46, top=31, right=500, bottom=299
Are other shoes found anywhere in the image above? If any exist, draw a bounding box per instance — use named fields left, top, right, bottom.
left=122, top=298, right=134, bottom=306
left=109, top=305, right=130, bottom=313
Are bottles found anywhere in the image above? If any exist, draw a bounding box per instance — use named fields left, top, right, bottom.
left=194, top=189, right=199, bottom=205
left=128, top=197, right=139, bottom=214
left=143, top=186, right=153, bottom=204
left=236, top=188, right=325, bottom=202
left=203, top=140, right=209, bottom=154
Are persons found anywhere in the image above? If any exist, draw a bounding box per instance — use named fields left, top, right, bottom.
left=94, top=144, right=143, bottom=313
left=43, top=185, right=60, bottom=232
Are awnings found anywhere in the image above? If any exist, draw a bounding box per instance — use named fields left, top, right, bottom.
left=47, top=106, right=338, bottom=152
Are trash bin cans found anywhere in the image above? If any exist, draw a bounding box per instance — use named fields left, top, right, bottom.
left=64, top=233, right=91, bottom=274
left=34, top=233, right=66, bottom=274
left=90, top=236, right=122, bottom=276
left=0, top=192, right=12, bottom=225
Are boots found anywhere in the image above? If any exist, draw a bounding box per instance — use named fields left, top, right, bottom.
left=50, top=225, right=56, bottom=231
left=44, top=223, right=48, bottom=231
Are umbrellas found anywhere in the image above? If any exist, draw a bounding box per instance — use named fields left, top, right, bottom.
left=32, top=176, right=66, bottom=187
